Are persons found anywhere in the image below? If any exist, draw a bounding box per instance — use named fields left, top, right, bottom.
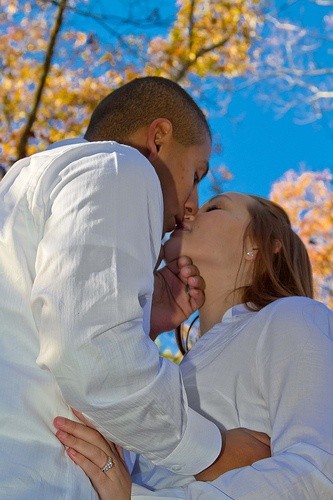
left=0, top=77, right=271, bottom=499
left=55, top=192, right=333, bottom=500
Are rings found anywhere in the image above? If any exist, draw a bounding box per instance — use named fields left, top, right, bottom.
left=100, top=456, right=114, bottom=473
